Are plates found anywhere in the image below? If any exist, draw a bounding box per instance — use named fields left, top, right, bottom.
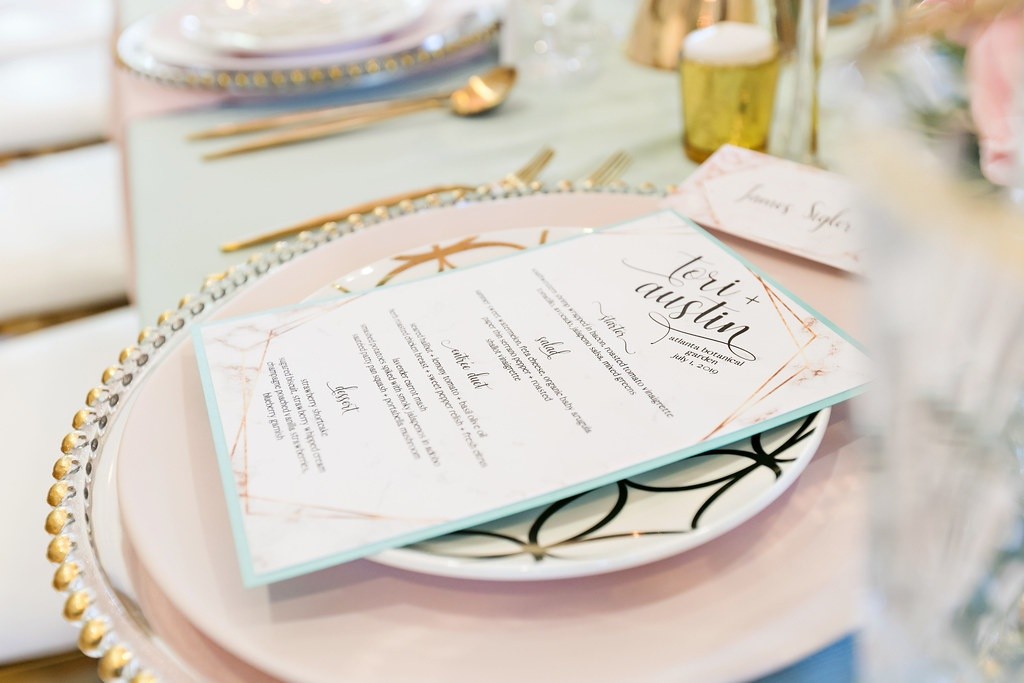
left=178, top=2, right=429, bottom=51
left=44, top=180, right=867, bottom=683
left=112, top=2, right=507, bottom=93
left=294, top=224, right=830, bottom=586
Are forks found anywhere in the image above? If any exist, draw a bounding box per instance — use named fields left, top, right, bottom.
left=221, top=144, right=553, bottom=254
left=572, top=151, right=631, bottom=187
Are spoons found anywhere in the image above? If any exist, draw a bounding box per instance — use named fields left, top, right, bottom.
left=204, top=67, right=517, bottom=158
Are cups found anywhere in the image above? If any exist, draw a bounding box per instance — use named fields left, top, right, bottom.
left=677, top=43, right=780, bottom=162
